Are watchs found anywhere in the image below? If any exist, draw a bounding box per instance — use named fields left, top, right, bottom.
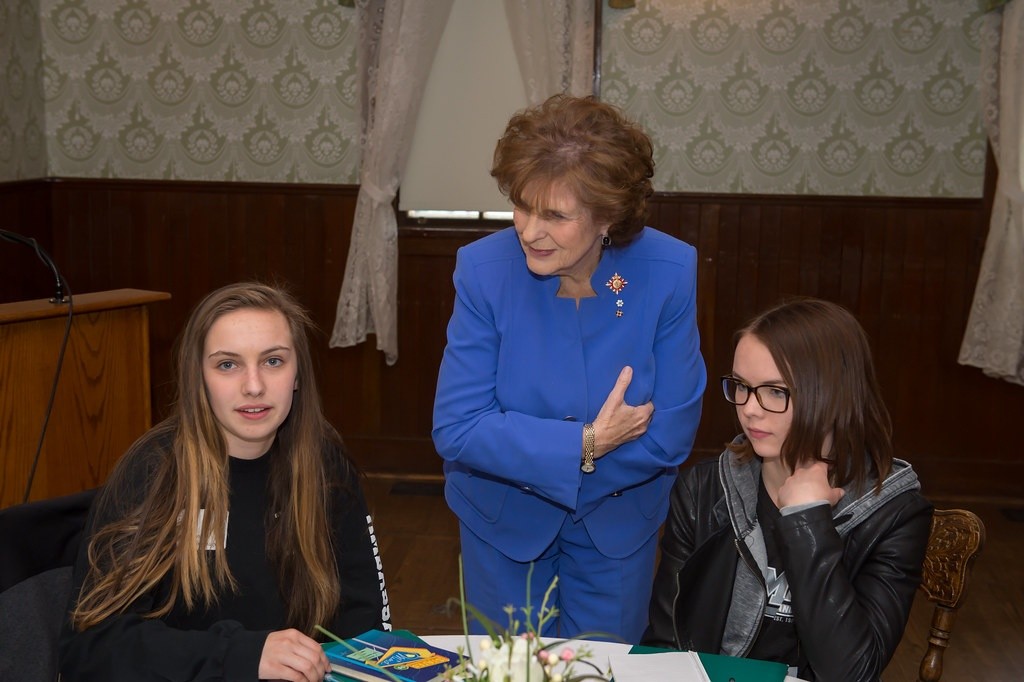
left=580, top=424, right=597, bottom=474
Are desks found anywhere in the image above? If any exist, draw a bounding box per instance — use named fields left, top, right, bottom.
left=318, top=634, right=802, bottom=682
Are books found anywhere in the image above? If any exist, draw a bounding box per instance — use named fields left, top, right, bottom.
left=311, top=629, right=474, bottom=682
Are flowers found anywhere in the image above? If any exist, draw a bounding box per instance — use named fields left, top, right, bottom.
left=438, top=550, right=606, bottom=682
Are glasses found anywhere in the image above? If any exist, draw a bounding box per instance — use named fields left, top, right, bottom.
left=719, top=373, right=791, bottom=413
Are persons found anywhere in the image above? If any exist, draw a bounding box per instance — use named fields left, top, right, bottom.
left=430, top=94, right=708, bottom=644
left=54, top=281, right=393, bottom=681
left=639, top=298, right=937, bottom=680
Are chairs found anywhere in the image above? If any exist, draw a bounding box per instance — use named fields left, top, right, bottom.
left=917, top=510, right=984, bottom=682
